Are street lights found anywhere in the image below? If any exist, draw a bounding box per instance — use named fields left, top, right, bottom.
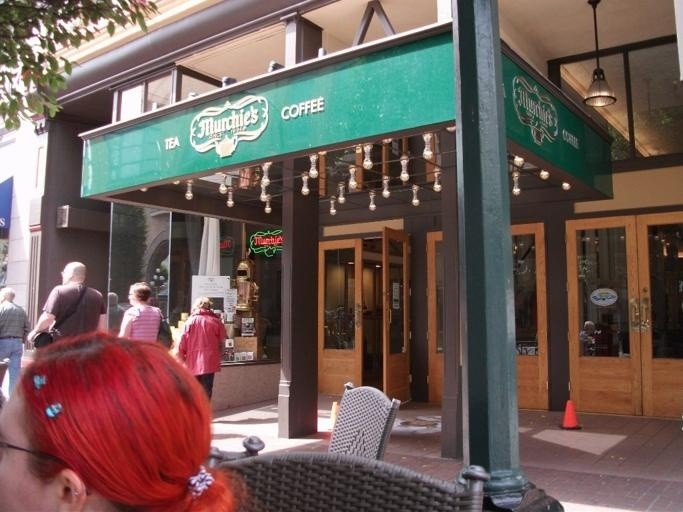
left=148, top=267, right=164, bottom=308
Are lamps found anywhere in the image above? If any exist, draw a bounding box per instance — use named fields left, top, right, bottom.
left=582, top=0, right=616, bottom=106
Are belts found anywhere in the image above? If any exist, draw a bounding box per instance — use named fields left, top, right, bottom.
left=0, top=336, right=19, bottom=339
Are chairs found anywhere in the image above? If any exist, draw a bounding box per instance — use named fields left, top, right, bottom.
left=208, top=382, right=489, bottom=512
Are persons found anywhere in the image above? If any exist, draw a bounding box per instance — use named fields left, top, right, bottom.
left=27, top=261, right=111, bottom=341
left=580, top=320, right=596, bottom=342
left=0, top=331, right=236, bottom=512
left=118, top=283, right=163, bottom=342
left=0, top=287, right=31, bottom=399
left=109, top=292, right=125, bottom=333
left=176, top=297, right=229, bottom=399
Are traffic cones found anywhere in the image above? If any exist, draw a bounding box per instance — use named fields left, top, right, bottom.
left=560, top=399, right=583, bottom=432
left=327, top=400, right=339, bottom=430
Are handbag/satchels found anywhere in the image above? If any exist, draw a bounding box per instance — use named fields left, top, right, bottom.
left=32, top=332, right=52, bottom=348
left=156, top=319, right=172, bottom=348
